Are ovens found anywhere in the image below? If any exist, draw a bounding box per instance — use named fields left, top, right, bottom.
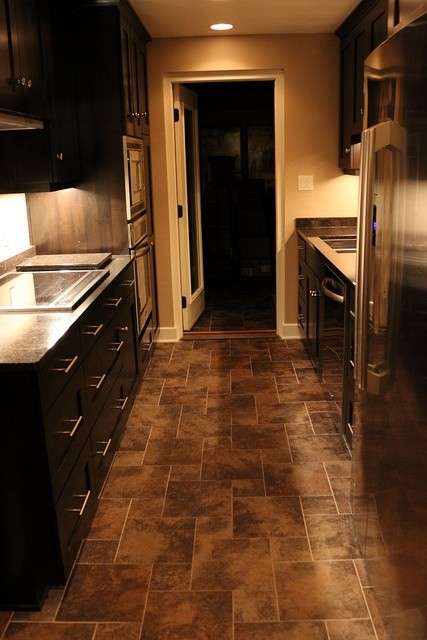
left=121, top=135, right=147, bottom=222
left=125, top=214, right=153, bottom=338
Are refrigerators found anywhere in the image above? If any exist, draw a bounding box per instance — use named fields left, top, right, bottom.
left=347, top=11, right=427, bottom=640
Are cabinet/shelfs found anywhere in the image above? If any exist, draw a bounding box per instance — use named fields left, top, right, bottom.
left=354, top=1, right=388, bottom=135
left=0, top=255, right=140, bottom=586
left=0, top=1, right=48, bottom=122
left=339, top=20, right=353, bottom=169
left=307, top=242, right=317, bottom=361
left=26, top=136, right=161, bottom=382
left=1, top=1, right=90, bottom=195
left=344, top=286, right=355, bottom=448
left=294, top=233, right=308, bottom=350
left=90, top=3, right=153, bottom=136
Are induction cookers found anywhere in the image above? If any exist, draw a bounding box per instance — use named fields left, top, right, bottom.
left=0, top=270, right=109, bottom=311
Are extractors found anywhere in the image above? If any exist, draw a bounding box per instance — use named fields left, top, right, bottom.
left=0, top=110, right=44, bottom=138
